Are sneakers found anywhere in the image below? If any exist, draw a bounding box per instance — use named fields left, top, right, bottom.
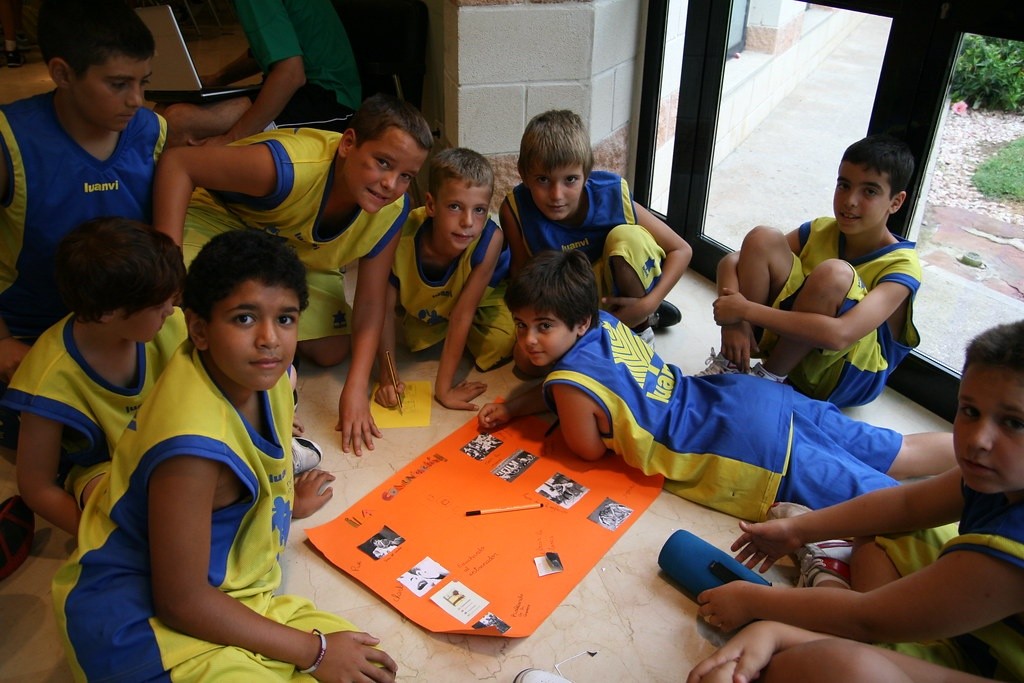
left=766, top=502, right=853, bottom=589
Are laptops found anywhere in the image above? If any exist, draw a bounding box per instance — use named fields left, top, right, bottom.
left=133, top=4, right=262, bottom=104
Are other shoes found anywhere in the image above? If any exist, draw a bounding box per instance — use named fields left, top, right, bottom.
left=287, top=436, right=321, bottom=475
left=515, top=669, right=572, bottom=683
left=647, top=300, right=681, bottom=331
left=0, top=496, right=36, bottom=583
left=745, top=362, right=788, bottom=382
left=694, top=350, right=740, bottom=376
left=639, top=328, right=655, bottom=352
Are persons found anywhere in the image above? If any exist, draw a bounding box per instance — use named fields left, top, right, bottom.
left=513, top=622, right=1001, bottom=683
left=702, top=136, right=920, bottom=404
left=499, top=110, right=693, bottom=337
left=399, top=570, right=450, bottom=597
left=153, top=97, right=436, bottom=457
left=478, top=250, right=959, bottom=525
left=551, top=483, right=573, bottom=501
left=0, top=0, right=168, bottom=451
left=157, top=0, right=362, bottom=146
left=371, top=536, right=397, bottom=558
left=483, top=615, right=507, bottom=634
left=52, top=229, right=398, bottom=683
left=374, top=149, right=518, bottom=409
left=0, top=222, right=320, bottom=541
left=698, top=322, right=1024, bottom=683
left=599, top=503, right=631, bottom=528
left=496, top=454, right=534, bottom=480
left=464, top=434, right=500, bottom=460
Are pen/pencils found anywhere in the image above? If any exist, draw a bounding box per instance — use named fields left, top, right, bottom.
left=386, top=351, right=404, bottom=414
left=465, top=503, right=543, bottom=517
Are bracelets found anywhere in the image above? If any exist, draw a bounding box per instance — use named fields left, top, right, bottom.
left=293, top=629, right=327, bottom=676
